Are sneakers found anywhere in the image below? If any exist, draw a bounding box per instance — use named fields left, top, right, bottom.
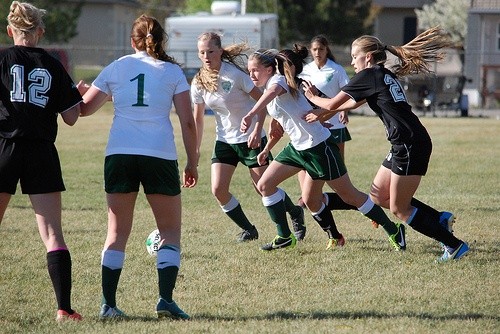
left=156, top=299, right=191, bottom=320
left=440, top=240, right=470, bottom=262
left=259, top=233, right=297, bottom=253
left=99, top=304, right=128, bottom=319
left=388, top=223, right=406, bottom=250
left=54, top=310, right=82, bottom=323
left=371, top=219, right=379, bottom=228
left=325, top=233, right=345, bottom=251
left=440, top=211, right=456, bottom=236
left=290, top=205, right=306, bottom=241
left=236, top=225, right=258, bottom=242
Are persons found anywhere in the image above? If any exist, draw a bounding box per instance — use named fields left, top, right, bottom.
left=239, top=49, right=406, bottom=254
left=0, top=1, right=84, bottom=321
left=277, top=47, right=360, bottom=212
left=296, top=36, right=352, bottom=187
left=301, top=25, right=474, bottom=262
left=81, top=16, right=199, bottom=320
left=190, top=32, right=306, bottom=241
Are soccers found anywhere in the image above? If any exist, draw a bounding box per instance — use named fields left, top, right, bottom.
left=146, top=228, right=162, bottom=258
